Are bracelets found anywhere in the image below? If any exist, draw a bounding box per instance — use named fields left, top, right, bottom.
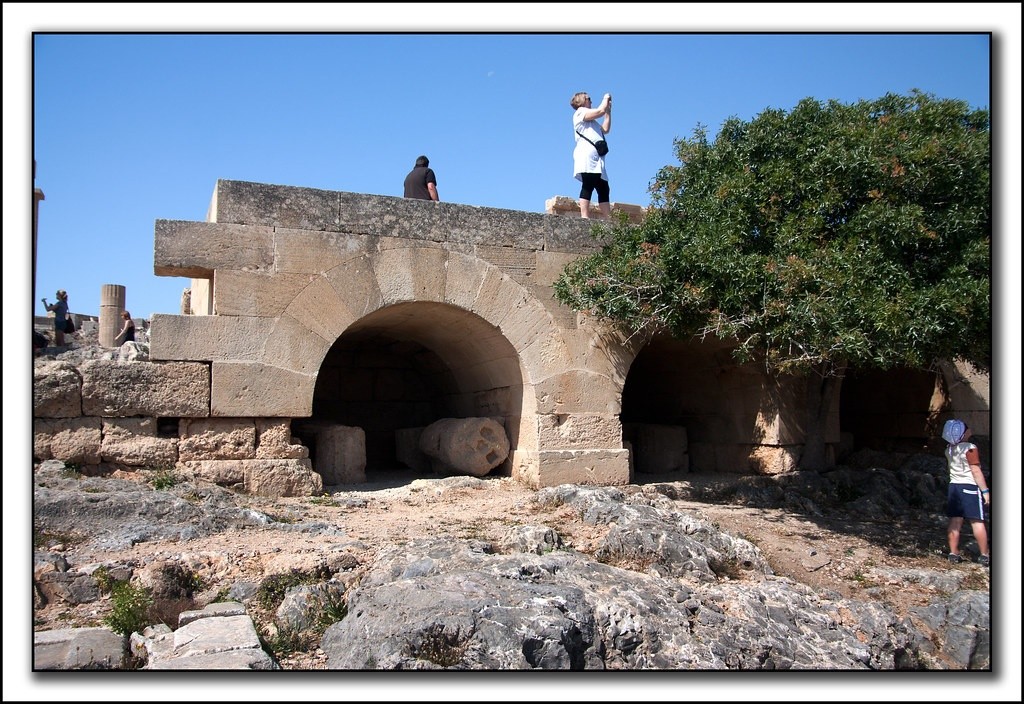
left=981, top=488, right=990, bottom=493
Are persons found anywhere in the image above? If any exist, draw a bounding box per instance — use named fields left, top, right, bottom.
left=568, top=91, right=614, bottom=223
left=42, top=289, right=68, bottom=347
left=403, top=155, right=439, bottom=202
left=942, top=420, right=991, bottom=567
left=114, top=310, right=136, bottom=345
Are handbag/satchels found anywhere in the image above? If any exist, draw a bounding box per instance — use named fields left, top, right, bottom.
left=595, top=140, right=608, bottom=157
left=62, top=317, right=74, bottom=334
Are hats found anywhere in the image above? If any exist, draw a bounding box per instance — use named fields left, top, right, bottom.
left=942, top=420, right=965, bottom=445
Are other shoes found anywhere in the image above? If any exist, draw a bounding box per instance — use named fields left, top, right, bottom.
left=977, top=554, right=989, bottom=567
left=948, top=555, right=963, bottom=564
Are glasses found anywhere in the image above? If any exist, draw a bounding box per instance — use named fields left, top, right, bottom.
left=585, top=98, right=591, bottom=101
